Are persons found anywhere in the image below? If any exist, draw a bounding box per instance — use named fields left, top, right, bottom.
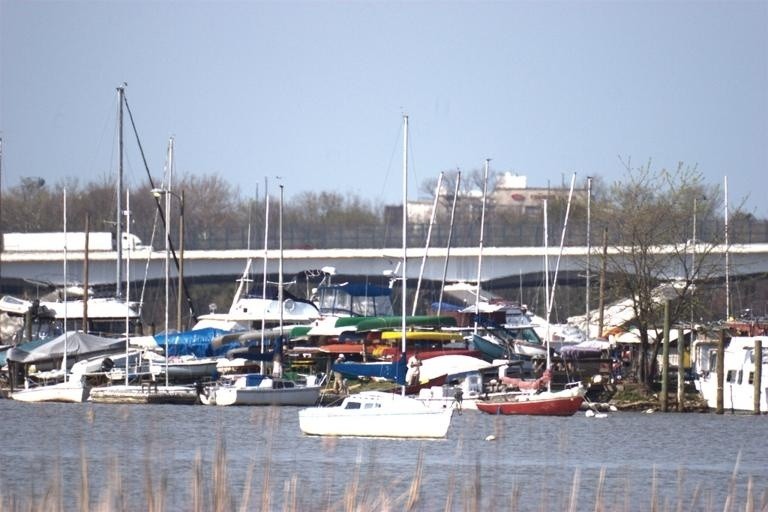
left=406, top=352, right=422, bottom=386
left=332, top=354, right=346, bottom=394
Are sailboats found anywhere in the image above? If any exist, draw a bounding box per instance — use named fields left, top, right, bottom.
left=12, top=184, right=87, bottom=404
left=294, top=112, right=459, bottom=439
left=89, top=83, right=203, bottom=404
left=685, top=173, right=767, bottom=414
left=475, top=197, right=587, bottom=416
left=211, top=182, right=324, bottom=408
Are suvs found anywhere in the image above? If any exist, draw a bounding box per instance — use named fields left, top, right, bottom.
left=640, top=348, right=697, bottom=395
